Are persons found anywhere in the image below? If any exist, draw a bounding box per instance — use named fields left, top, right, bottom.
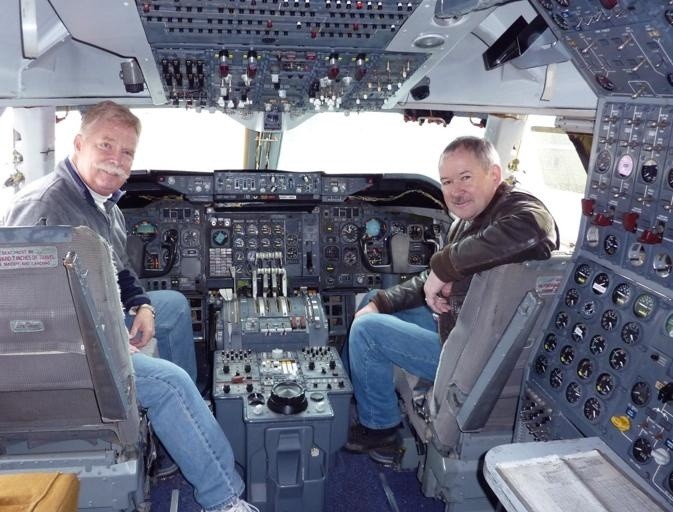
left=340, top=136, right=560, bottom=465
left=0, top=100, right=259, bottom=512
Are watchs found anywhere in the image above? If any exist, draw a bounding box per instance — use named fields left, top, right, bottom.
left=136, top=304, right=156, bottom=318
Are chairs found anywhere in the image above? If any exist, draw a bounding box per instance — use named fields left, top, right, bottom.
left=395, top=251, right=571, bottom=501
left=0, top=225, right=155, bottom=478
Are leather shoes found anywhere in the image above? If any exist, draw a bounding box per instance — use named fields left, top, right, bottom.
left=341, top=422, right=403, bottom=464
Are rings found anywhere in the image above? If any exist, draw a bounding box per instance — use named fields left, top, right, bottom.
left=425, top=297, right=428, bottom=301
